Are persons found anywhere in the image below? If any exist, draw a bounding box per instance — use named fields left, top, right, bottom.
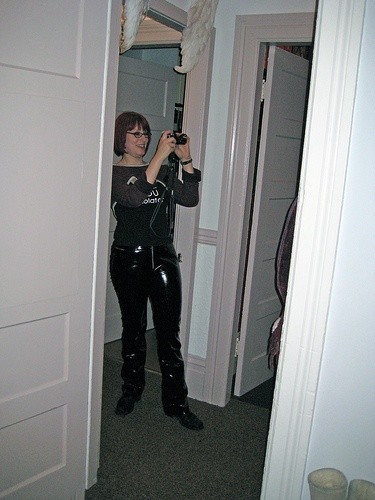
left=109, top=112, right=205, bottom=431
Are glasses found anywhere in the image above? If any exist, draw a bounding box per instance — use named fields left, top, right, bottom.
left=127, top=131, right=152, bottom=138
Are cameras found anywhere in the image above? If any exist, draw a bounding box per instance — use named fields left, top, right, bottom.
left=167, top=130, right=187, bottom=163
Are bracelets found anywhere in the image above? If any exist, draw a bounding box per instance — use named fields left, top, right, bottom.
left=180, top=158, right=192, bottom=166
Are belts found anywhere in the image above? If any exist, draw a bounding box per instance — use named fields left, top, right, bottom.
left=113, top=244, right=175, bottom=254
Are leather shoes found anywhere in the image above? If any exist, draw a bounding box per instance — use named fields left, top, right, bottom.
left=115, top=382, right=145, bottom=415
left=163, top=400, right=204, bottom=431
